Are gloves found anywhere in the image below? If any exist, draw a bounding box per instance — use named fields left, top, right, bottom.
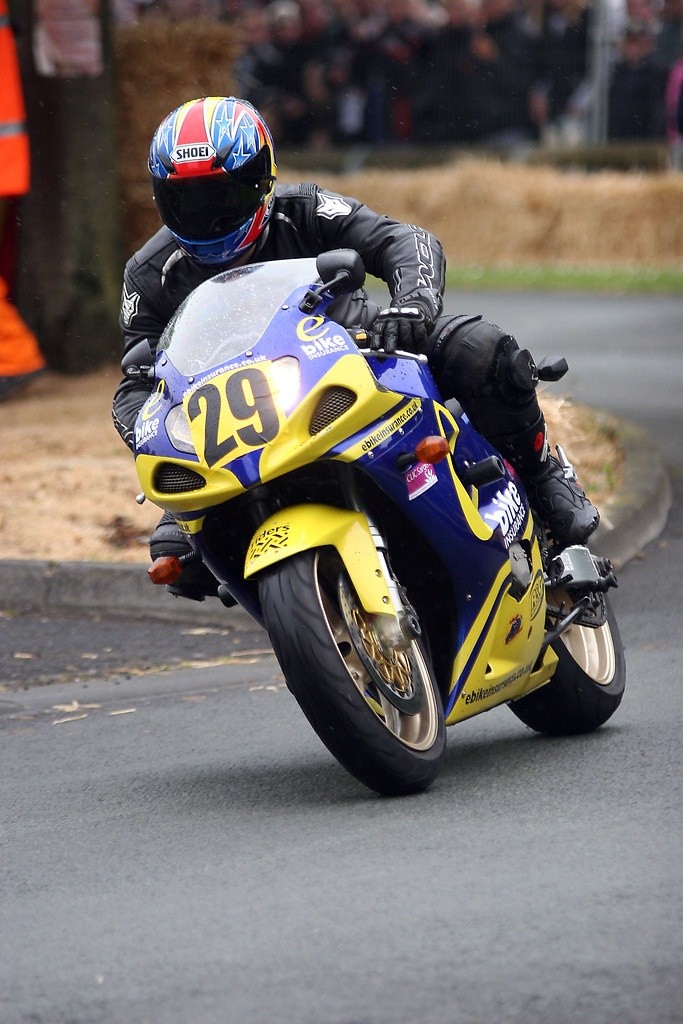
left=371, top=300, right=434, bottom=354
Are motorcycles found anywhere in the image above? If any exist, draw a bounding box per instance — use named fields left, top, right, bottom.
left=117, top=246, right=632, bottom=794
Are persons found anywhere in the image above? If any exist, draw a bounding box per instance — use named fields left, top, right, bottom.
left=108, top=93, right=598, bottom=593
left=0, top=0, right=683, bottom=400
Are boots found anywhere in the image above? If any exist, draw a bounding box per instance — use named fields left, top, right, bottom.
left=478, top=409, right=599, bottom=548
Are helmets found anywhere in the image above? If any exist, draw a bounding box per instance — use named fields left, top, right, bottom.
left=146, top=95, right=277, bottom=275
